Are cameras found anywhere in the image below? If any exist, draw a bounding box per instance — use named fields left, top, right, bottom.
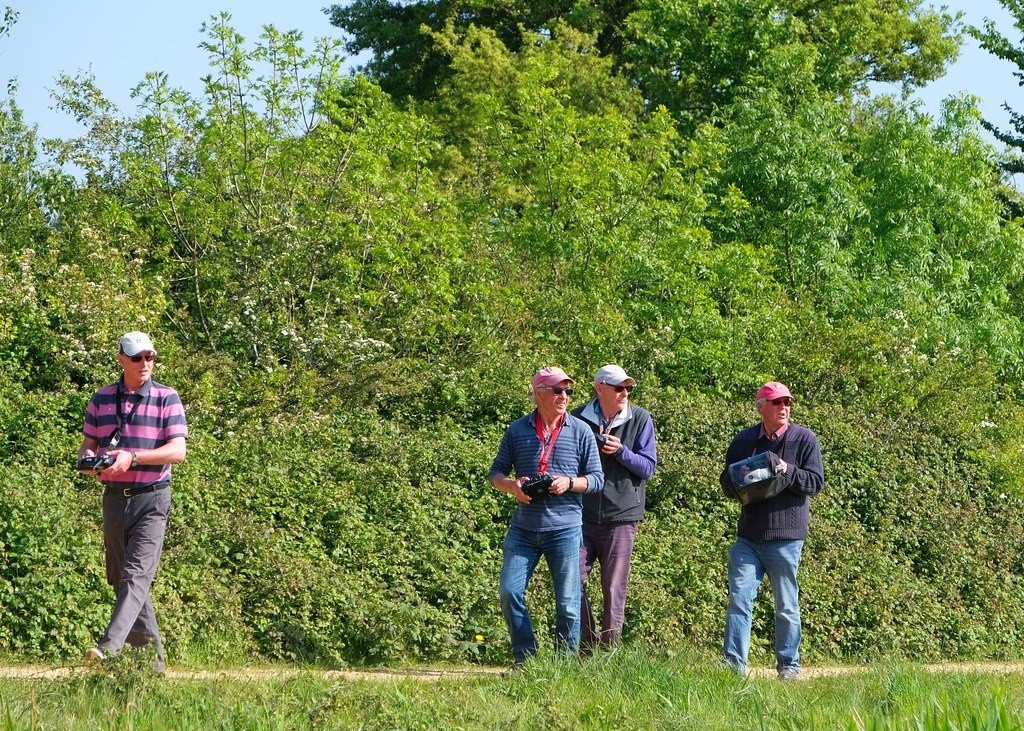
left=522, top=471, right=556, bottom=505
left=74, top=456, right=114, bottom=476
left=593, top=432, right=607, bottom=451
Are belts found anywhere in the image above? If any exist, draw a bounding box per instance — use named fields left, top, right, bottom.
left=108, top=482, right=169, bottom=498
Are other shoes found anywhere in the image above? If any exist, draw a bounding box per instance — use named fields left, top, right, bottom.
left=85, top=648, right=110, bottom=676
left=779, top=668, right=798, bottom=681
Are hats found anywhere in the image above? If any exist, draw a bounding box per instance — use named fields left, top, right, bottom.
left=757, top=382, right=795, bottom=401
left=594, top=365, right=635, bottom=386
left=532, top=367, right=574, bottom=390
left=118, top=331, right=157, bottom=357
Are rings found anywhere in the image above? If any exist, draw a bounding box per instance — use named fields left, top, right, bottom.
left=557, top=489, right=559, bottom=492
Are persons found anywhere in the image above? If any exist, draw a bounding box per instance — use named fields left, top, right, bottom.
left=486, top=364, right=604, bottom=669
left=571, top=364, right=657, bottom=657
left=77, top=331, right=188, bottom=674
left=719, top=381, right=823, bottom=679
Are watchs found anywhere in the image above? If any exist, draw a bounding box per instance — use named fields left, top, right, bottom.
left=131, top=451, right=138, bottom=467
left=567, top=476, right=574, bottom=489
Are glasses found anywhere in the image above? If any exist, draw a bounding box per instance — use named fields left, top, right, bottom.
left=123, top=352, right=155, bottom=363
left=766, top=398, right=792, bottom=407
left=538, top=386, right=574, bottom=396
left=600, top=382, right=633, bottom=393
